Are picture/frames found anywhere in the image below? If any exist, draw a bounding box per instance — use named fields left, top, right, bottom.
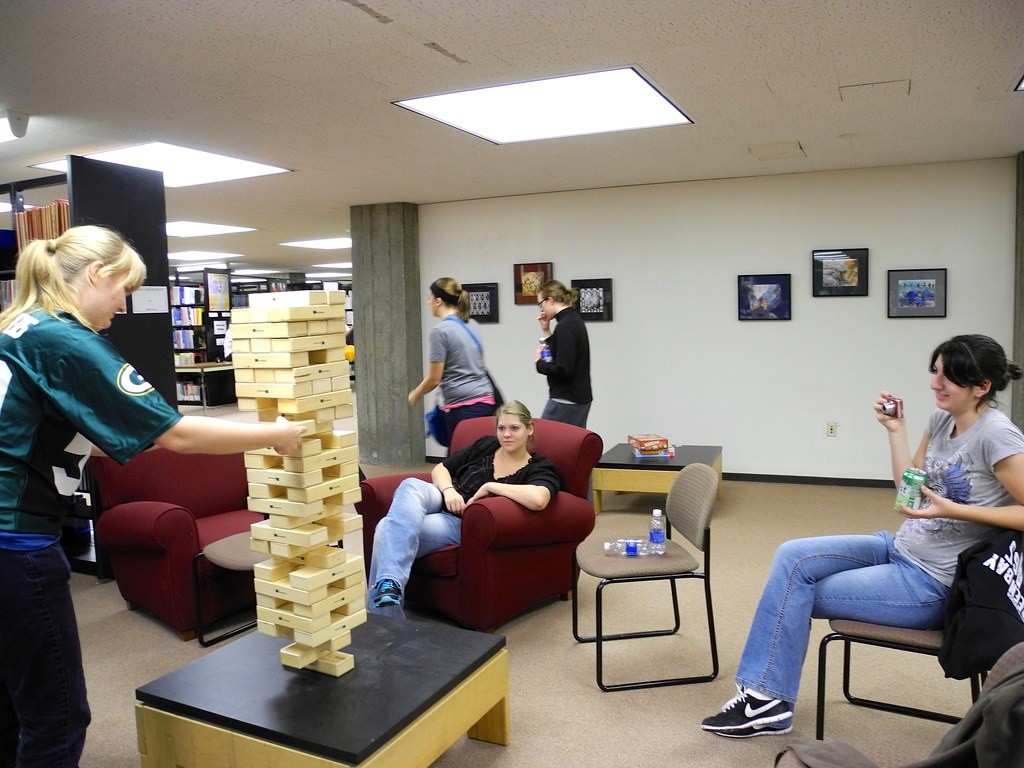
left=887, top=268, right=947, bottom=318
left=570, top=278, right=612, bottom=321
left=460, top=283, right=498, bottom=323
left=812, top=248, right=868, bottom=297
left=513, top=262, right=553, bottom=305
left=737, top=274, right=791, bottom=320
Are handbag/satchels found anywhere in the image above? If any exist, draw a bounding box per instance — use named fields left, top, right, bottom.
left=425, top=373, right=503, bottom=447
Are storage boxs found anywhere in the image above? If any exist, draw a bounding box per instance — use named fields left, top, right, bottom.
left=230, top=289, right=368, bottom=676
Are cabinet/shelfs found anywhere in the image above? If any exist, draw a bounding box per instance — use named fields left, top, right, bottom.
left=0, top=152, right=355, bottom=579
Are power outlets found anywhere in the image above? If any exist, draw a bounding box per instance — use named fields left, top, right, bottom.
left=826, top=422, right=837, bottom=436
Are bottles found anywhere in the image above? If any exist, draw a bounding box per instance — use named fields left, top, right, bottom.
left=604, top=539, right=649, bottom=557
left=539, top=338, right=552, bottom=362
left=647, top=509, right=666, bottom=555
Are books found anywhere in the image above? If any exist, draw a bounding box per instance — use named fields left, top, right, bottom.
left=15, top=198, right=70, bottom=254
left=170, top=286, right=204, bottom=401
left=0, top=280, right=17, bottom=310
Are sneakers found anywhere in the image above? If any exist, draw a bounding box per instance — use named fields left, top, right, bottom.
left=715, top=724, right=793, bottom=737
left=372, top=579, right=403, bottom=608
left=701, top=681, right=793, bottom=730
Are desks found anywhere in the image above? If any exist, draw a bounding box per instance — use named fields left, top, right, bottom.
left=591, top=443, right=722, bottom=515
left=174, top=361, right=233, bottom=415
left=134, top=612, right=510, bottom=767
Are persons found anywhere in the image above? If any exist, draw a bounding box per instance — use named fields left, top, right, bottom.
left=535, top=280, right=593, bottom=429
left=408, top=277, right=496, bottom=432
left=366, top=400, right=560, bottom=620
left=0, top=225, right=308, bottom=768
left=703, top=335, right=1024, bottom=739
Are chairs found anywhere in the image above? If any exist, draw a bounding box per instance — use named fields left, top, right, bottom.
left=193, top=512, right=348, bottom=646
left=570, top=462, right=721, bottom=692
left=817, top=530, right=1024, bottom=740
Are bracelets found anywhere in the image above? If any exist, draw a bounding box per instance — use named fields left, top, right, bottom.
left=441, top=487, right=453, bottom=492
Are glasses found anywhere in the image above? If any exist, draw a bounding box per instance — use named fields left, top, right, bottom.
left=537, top=296, right=550, bottom=310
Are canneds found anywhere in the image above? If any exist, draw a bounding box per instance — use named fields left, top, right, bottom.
left=894, top=467, right=927, bottom=512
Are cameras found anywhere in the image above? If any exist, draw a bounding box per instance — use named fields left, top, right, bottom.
left=882, top=397, right=904, bottom=419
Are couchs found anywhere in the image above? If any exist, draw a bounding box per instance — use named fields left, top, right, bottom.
left=355, top=417, right=603, bottom=633
left=87, top=443, right=366, bottom=641
